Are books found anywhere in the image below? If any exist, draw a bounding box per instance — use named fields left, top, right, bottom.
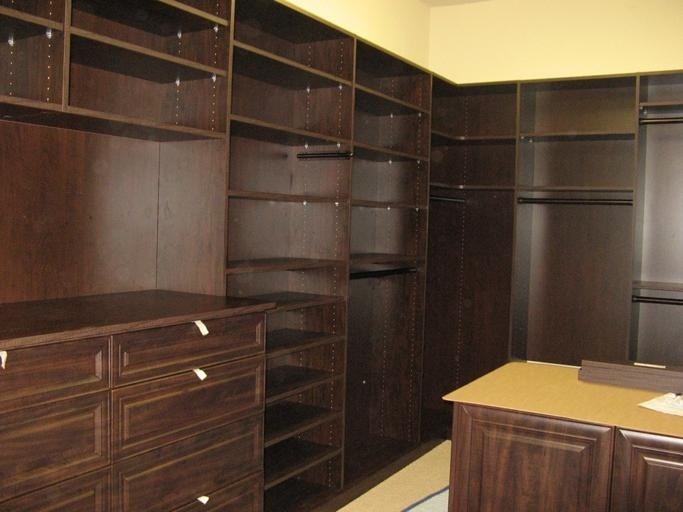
left=635, top=390, right=683, bottom=417
left=577, top=356, right=682, bottom=395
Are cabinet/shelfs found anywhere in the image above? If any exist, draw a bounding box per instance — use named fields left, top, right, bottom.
left=287, top=28, right=429, bottom=512
left=158, top=0, right=361, bottom=509
left=507, top=71, right=646, bottom=368
left=625, top=67, right=681, bottom=369
left=0, top=0, right=231, bottom=139
left=426, top=66, right=532, bottom=452
left=0, top=285, right=280, bottom=511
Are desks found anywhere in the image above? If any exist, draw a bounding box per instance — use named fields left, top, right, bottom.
left=445, top=361, right=682, bottom=511
